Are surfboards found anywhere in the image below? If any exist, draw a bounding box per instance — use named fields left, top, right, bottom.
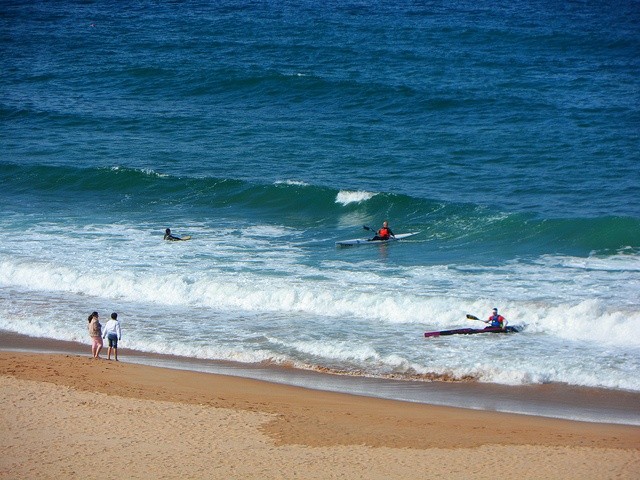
left=180, top=235, right=191, bottom=240
left=335, top=232, right=421, bottom=244
left=424, top=324, right=527, bottom=338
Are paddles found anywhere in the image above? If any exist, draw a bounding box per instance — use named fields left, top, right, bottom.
left=466, top=314, right=495, bottom=326
left=363, top=225, right=377, bottom=235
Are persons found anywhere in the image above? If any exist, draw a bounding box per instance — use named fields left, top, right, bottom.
left=484, top=308, right=508, bottom=330
left=368, top=222, right=395, bottom=241
left=103, top=313, right=120, bottom=361
left=88, top=312, right=103, bottom=358
left=164, top=228, right=181, bottom=241
